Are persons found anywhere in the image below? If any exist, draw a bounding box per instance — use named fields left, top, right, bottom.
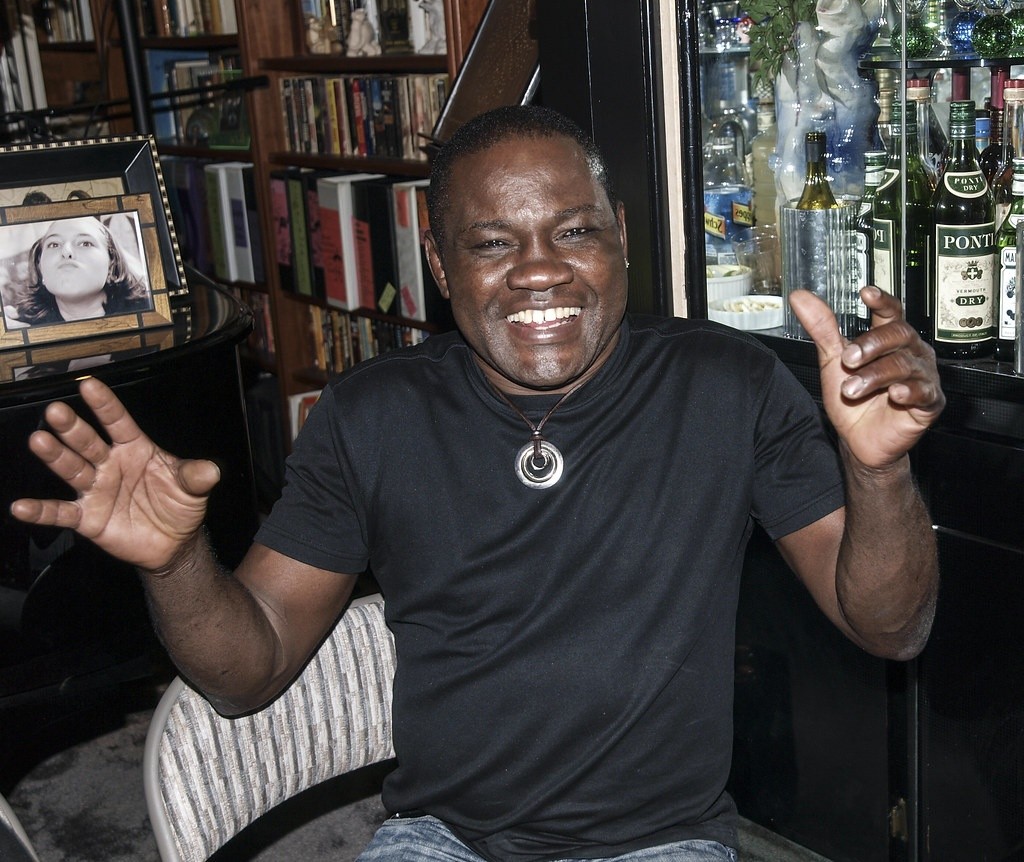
left=12, top=216, right=149, bottom=326
left=11, top=105, right=947, bottom=862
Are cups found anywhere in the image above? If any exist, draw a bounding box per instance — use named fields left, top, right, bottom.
left=777, top=193, right=863, bottom=343
left=728, top=223, right=782, bottom=297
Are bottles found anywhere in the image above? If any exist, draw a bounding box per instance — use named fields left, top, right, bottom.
left=687, top=0, right=1024, bottom=378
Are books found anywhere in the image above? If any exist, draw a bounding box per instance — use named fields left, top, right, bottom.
left=45, top=0, right=94, bottom=42
left=303, top=0, right=431, bottom=54
left=137, top=0, right=238, bottom=38
left=222, top=284, right=428, bottom=441
left=280, top=74, right=449, bottom=161
left=160, top=155, right=434, bottom=321
left=146, top=50, right=242, bottom=139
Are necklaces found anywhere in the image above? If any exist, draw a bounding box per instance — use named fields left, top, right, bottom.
left=487, top=367, right=603, bottom=489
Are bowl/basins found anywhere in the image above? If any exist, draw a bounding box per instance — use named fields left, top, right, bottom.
left=705, top=263, right=753, bottom=304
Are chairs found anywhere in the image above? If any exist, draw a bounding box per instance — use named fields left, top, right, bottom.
left=144, top=595, right=398, bottom=862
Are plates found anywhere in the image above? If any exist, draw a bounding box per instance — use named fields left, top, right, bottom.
left=708, top=295, right=784, bottom=332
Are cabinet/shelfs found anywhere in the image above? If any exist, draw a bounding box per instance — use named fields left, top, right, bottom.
left=0, top=0, right=541, bottom=529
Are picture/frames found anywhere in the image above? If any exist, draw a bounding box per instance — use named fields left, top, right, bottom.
left=0, top=132, right=189, bottom=297
left=0, top=192, right=172, bottom=353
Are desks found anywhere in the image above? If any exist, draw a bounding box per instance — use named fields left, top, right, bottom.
left=0, top=273, right=265, bottom=549
